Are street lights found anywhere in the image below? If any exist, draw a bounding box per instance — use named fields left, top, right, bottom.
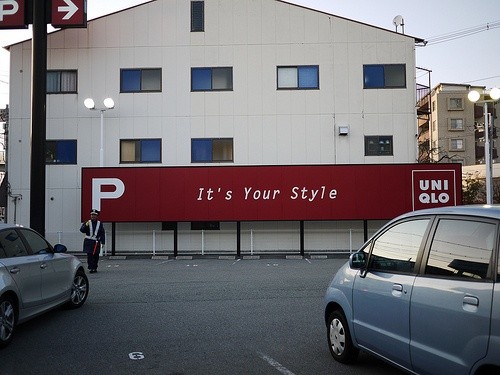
left=468, top=86, right=500, bottom=203
left=83, top=97, right=115, bottom=258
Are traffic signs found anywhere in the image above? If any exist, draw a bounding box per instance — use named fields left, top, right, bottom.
left=43, top=0, right=90, bottom=31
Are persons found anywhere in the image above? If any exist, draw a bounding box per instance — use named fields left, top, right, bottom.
left=80, top=209, right=105, bottom=273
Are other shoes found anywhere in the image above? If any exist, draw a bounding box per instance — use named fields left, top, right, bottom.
left=93, top=269, right=97, bottom=273
left=89, top=269, right=93, bottom=273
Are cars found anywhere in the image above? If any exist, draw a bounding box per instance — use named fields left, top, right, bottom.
left=323, top=204, right=500, bottom=375
left=0, top=223, right=89, bottom=346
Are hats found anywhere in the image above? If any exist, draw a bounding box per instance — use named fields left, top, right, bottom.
left=90, top=209, right=99, bottom=215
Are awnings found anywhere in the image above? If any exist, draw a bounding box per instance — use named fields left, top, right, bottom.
left=0, top=171, right=8, bottom=208
